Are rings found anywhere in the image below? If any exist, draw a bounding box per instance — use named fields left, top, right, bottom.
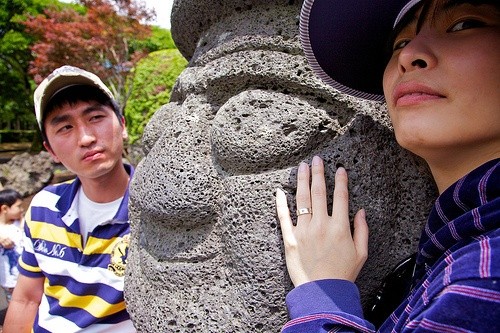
left=296, top=208, right=312, bottom=216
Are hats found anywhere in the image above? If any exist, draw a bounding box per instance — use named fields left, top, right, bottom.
left=33, top=65, right=120, bottom=132
left=299, top=0, right=421, bottom=103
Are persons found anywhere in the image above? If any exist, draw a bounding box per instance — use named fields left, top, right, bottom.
left=2, top=65, right=135, bottom=333
left=0, top=189, right=26, bottom=330
left=276, top=0, right=499, bottom=333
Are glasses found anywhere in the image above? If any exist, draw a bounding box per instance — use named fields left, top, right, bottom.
left=366, top=253, right=418, bottom=320
